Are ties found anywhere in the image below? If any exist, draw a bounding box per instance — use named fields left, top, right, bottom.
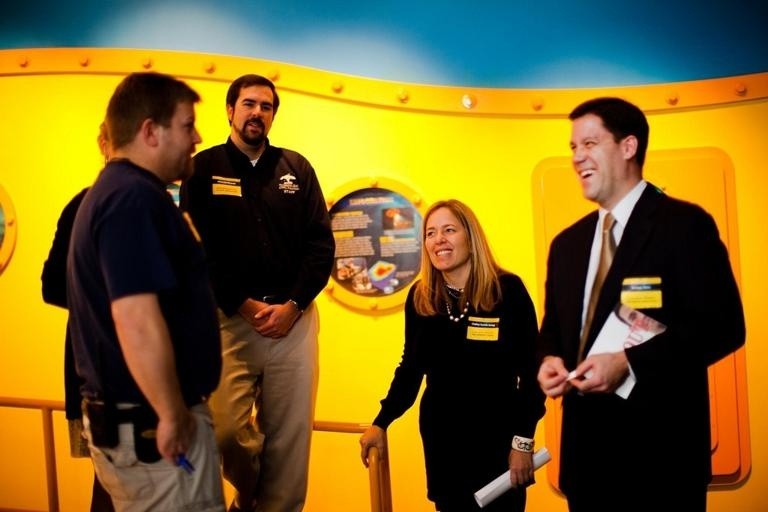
left=576, top=212, right=620, bottom=367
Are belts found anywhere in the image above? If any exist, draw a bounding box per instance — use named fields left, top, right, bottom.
left=249, top=293, right=290, bottom=306
left=78, top=393, right=209, bottom=426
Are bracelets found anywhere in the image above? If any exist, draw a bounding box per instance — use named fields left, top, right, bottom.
left=290, top=297, right=306, bottom=313
left=510, top=435, right=536, bottom=452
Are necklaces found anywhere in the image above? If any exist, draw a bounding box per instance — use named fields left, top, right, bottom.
left=442, top=294, right=471, bottom=326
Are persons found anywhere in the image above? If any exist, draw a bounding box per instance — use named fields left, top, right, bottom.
left=527, top=96, right=743, bottom=512
left=365, top=198, right=542, bottom=512
left=36, top=119, right=127, bottom=512
left=183, top=74, right=337, bottom=512
left=65, top=71, right=243, bottom=511
